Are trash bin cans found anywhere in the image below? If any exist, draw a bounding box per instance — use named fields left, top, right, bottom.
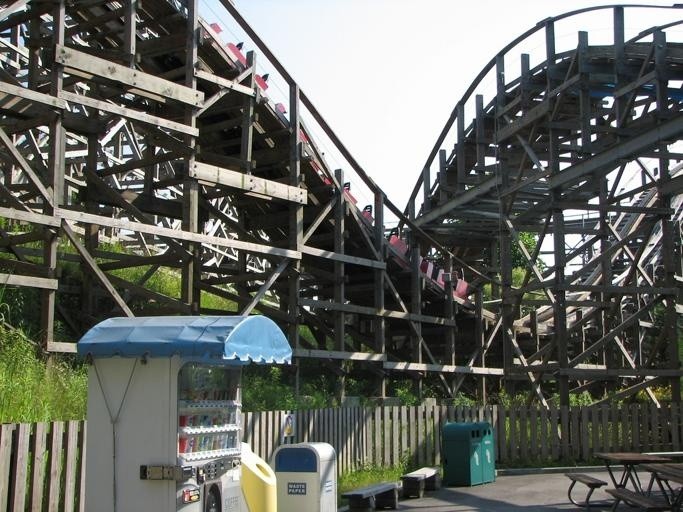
left=443, top=422, right=495, bottom=487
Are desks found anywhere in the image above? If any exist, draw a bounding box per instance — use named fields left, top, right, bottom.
left=594, top=452, right=672, bottom=498
left=639, top=463, right=683, bottom=512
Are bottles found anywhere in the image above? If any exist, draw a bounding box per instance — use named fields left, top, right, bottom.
left=180, top=372, right=238, bottom=452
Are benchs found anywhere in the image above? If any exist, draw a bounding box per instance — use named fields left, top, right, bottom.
left=400, top=466, right=441, bottom=498
left=605, top=488, right=673, bottom=512
left=341, top=483, right=399, bottom=512
left=564, top=474, right=607, bottom=507
